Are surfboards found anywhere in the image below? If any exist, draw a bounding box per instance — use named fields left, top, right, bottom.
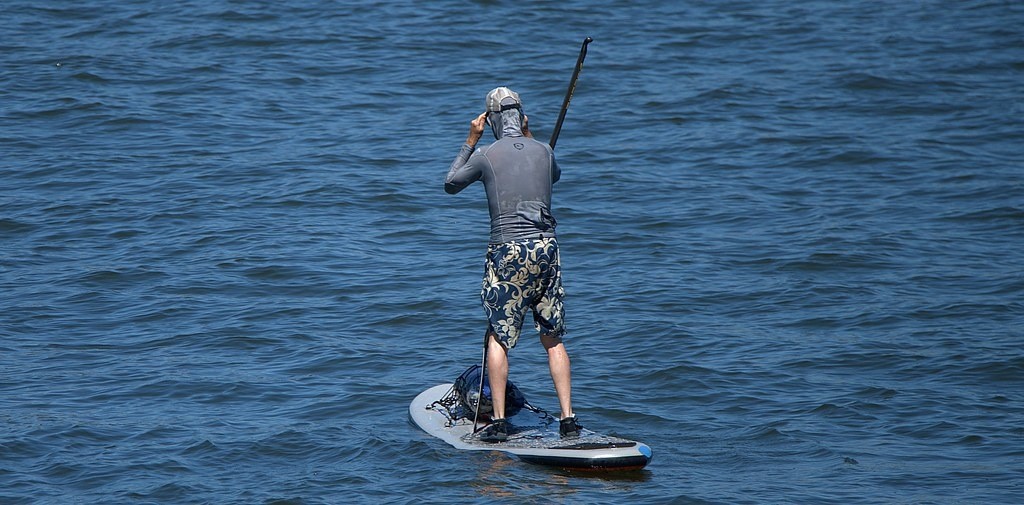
left=407, top=379, right=653, bottom=469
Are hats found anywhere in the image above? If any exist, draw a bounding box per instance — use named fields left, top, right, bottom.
left=485, top=87, right=522, bottom=115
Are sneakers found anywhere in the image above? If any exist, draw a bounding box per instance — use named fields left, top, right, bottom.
left=480, top=416, right=507, bottom=442
left=559, top=413, right=580, bottom=440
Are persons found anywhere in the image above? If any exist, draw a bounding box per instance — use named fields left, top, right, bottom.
left=444, top=86, right=583, bottom=442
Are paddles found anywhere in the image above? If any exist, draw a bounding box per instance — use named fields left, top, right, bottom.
left=472, top=33, right=594, bottom=435
